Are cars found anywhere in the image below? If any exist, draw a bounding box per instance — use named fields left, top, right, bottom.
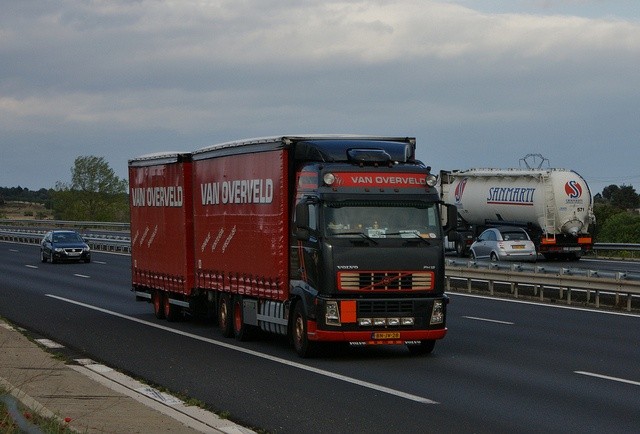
left=40, top=230, right=90, bottom=263
left=468, top=226, right=537, bottom=263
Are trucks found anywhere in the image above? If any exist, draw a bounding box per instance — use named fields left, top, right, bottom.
left=434, top=168, right=596, bottom=262
left=128, top=136, right=457, bottom=359
